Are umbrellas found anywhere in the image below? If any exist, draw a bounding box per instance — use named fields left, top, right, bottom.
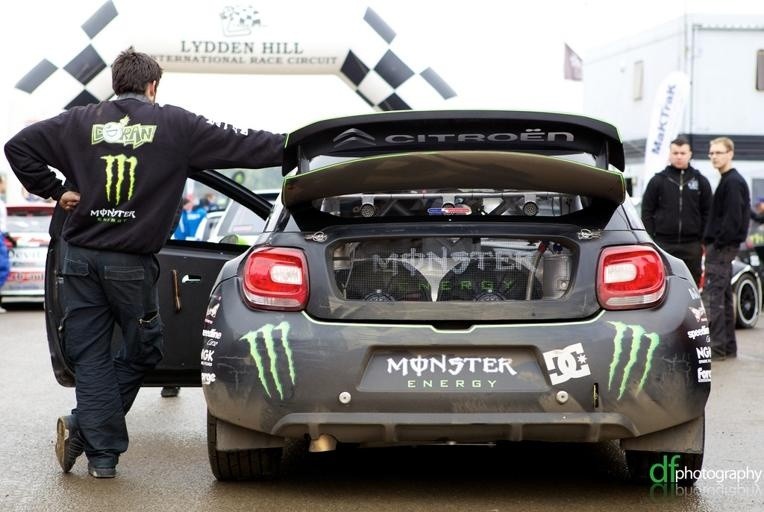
left=711, top=350, right=736, bottom=361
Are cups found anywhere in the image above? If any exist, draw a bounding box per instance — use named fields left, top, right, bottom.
left=709, top=151, right=727, bottom=156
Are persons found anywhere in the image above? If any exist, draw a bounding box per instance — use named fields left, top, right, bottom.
left=0, top=193, right=220, bottom=316
left=5, top=49, right=288, bottom=479
left=643, top=137, right=764, bottom=360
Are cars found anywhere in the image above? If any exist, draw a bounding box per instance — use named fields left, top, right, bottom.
left=0, top=200, right=762, bottom=338
left=41, top=106, right=713, bottom=494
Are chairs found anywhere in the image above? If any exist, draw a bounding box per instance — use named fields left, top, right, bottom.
left=161, top=387, right=179, bottom=397
left=54, top=414, right=84, bottom=474
left=87, top=456, right=117, bottom=479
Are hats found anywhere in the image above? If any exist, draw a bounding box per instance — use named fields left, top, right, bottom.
left=41, top=106, right=713, bottom=494
